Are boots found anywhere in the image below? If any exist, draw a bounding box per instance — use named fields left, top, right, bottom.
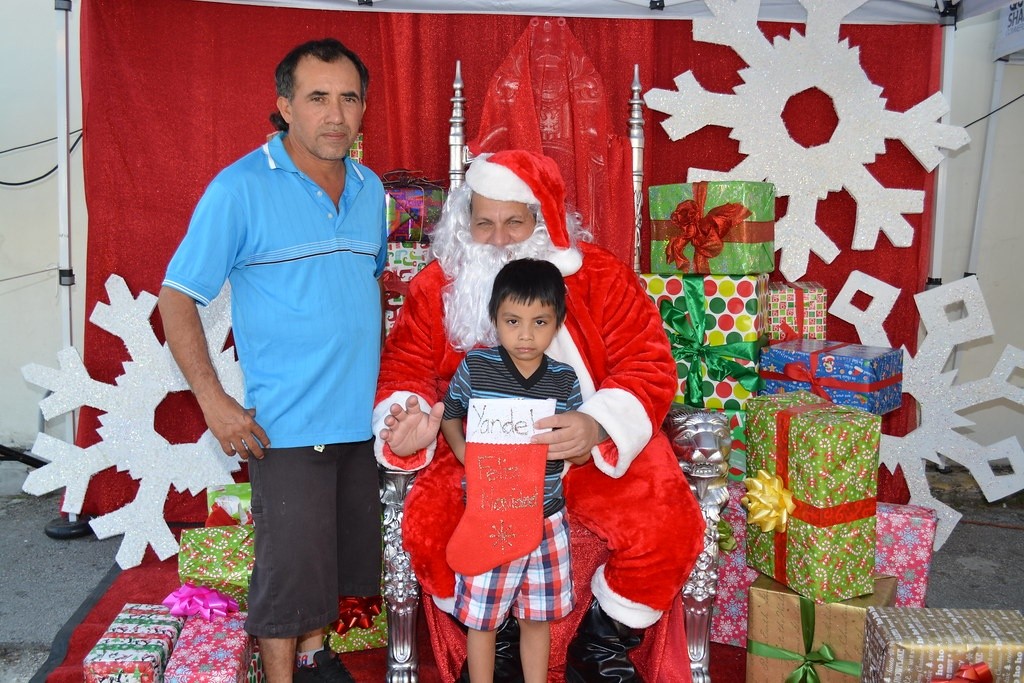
left=566, top=559, right=665, bottom=683
left=432, top=593, right=526, bottom=683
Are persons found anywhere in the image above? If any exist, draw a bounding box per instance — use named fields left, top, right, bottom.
left=374, top=149, right=706, bottom=683
left=441, top=258, right=591, bottom=683
left=159, top=38, right=388, bottom=683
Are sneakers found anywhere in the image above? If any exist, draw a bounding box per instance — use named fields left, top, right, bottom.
left=296, top=633, right=357, bottom=683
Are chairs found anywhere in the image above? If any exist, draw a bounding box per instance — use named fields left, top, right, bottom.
left=381, top=58, right=735, bottom=683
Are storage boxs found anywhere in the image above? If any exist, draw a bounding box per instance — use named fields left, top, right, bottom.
left=80, top=168, right=1023, bottom=683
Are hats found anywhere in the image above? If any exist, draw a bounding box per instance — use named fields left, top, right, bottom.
left=465, top=150, right=583, bottom=277
